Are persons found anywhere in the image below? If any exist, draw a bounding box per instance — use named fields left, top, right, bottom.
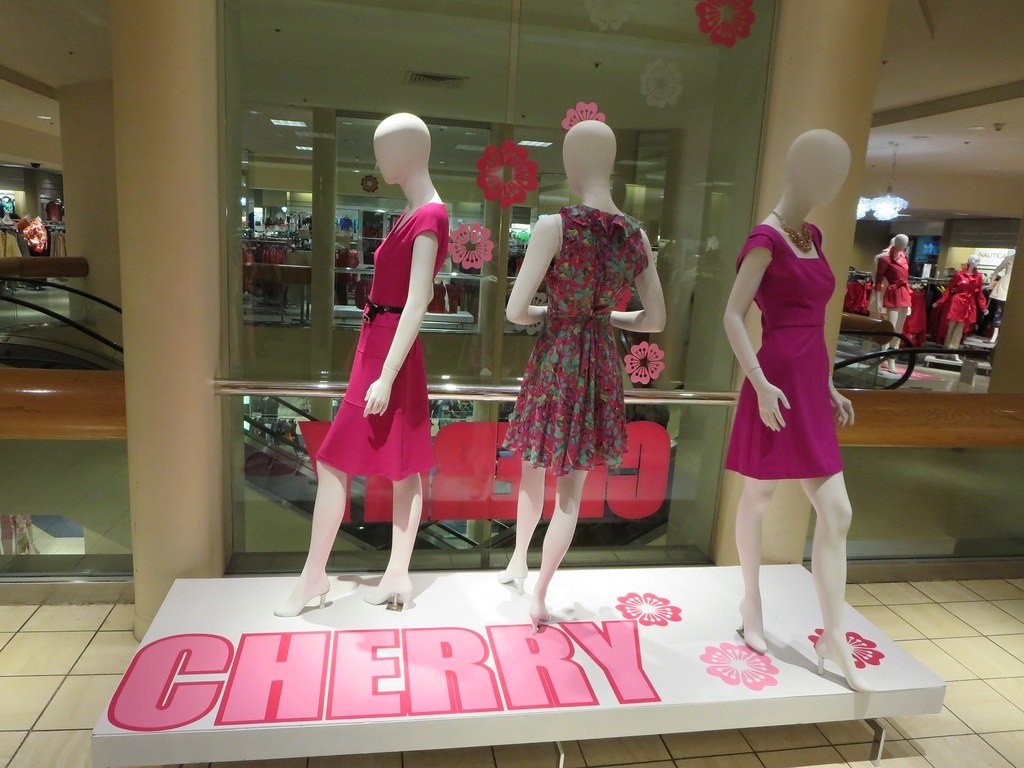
left=989, top=245, right=1016, bottom=342
left=272, top=112, right=451, bottom=618
left=723, top=128, right=877, bottom=696
left=498, top=118, right=668, bottom=633
left=0, top=202, right=22, bottom=257
left=869, top=233, right=912, bottom=375
left=931, top=255, right=989, bottom=360
